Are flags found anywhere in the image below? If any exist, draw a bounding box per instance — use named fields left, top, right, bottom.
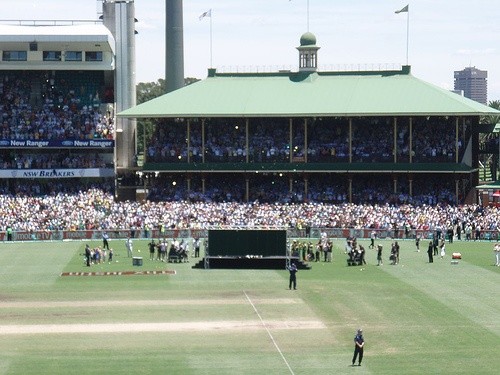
left=198, top=9, right=211, bottom=21
left=395, top=5, right=408, bottom=13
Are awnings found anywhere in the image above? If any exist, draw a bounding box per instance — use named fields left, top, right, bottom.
left=116, top=73, right=500, bottom=118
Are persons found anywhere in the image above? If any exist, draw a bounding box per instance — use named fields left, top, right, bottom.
left=352, top=328, right=365, bottom=367
left=1, top=73, right=500, bottom=290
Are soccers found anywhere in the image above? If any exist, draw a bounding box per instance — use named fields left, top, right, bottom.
left=359, top=268, right=363, bottom=271
left=138, top=249, right=141, bottom=253
